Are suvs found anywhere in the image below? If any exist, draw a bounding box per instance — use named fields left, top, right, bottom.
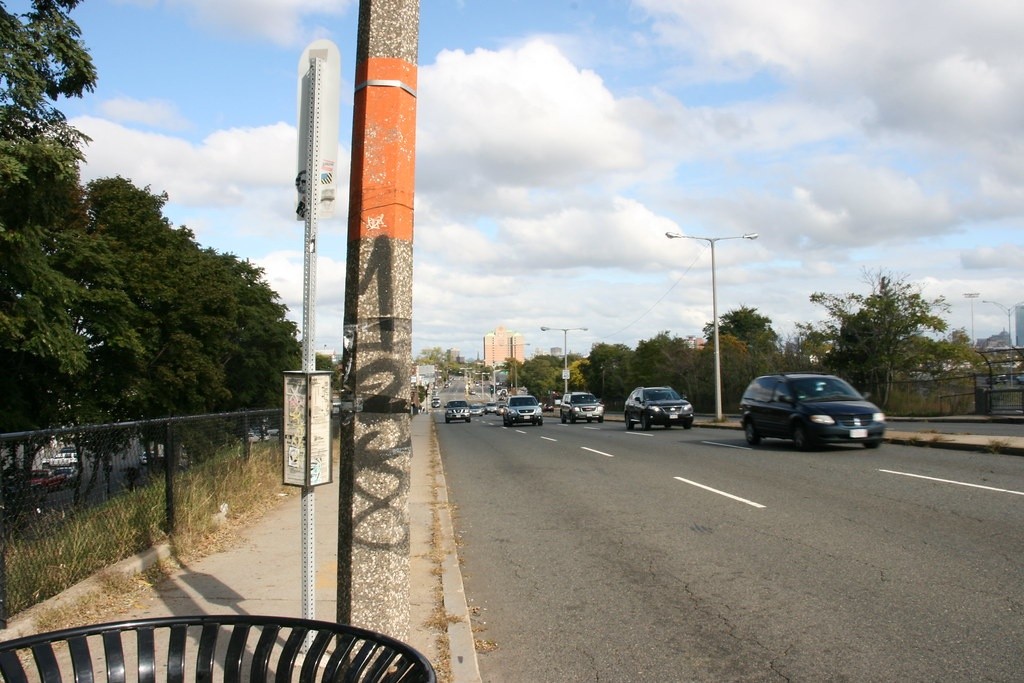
left=502, top=395, right=543, bottom=427
left=624, top=386, right=694, bottom=431
left=559, top=392, right=604, bottom=424
left=444, top=400, right=471, bottom=424
left=738, top=372, right=886, bottom=452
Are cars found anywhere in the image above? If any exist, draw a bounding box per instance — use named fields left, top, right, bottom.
left=247, top=427, right=280, bottom=443
left=444, top=375, right=455, bottom=388
left=430, top=390, right=441, bottom=408
left=985, top=374, right=1007, bottom=385
left=21, top=446, right=79, bottom=494
left=542, top=404, right=554, bottom=413
left=138, top=450, right=155, bottom=464
left=471, top=390, right=475, bottom=396
left=469, top=388, right=510, bottom=417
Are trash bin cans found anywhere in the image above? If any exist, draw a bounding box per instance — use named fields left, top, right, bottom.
left=975, top=385, right=1000, bottom=415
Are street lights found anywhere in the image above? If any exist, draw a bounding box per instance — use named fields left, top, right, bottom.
left=474, top=373, right=491, bottom=399
left=962, top=293, right=980, bottom=343
left=540, top=326, right=588, bottom=394
left=499, top=344, right=531, bottom=395
left=982, top=300, right=1024, bottom=387
left=665, top=231, right=759, bottom=421
